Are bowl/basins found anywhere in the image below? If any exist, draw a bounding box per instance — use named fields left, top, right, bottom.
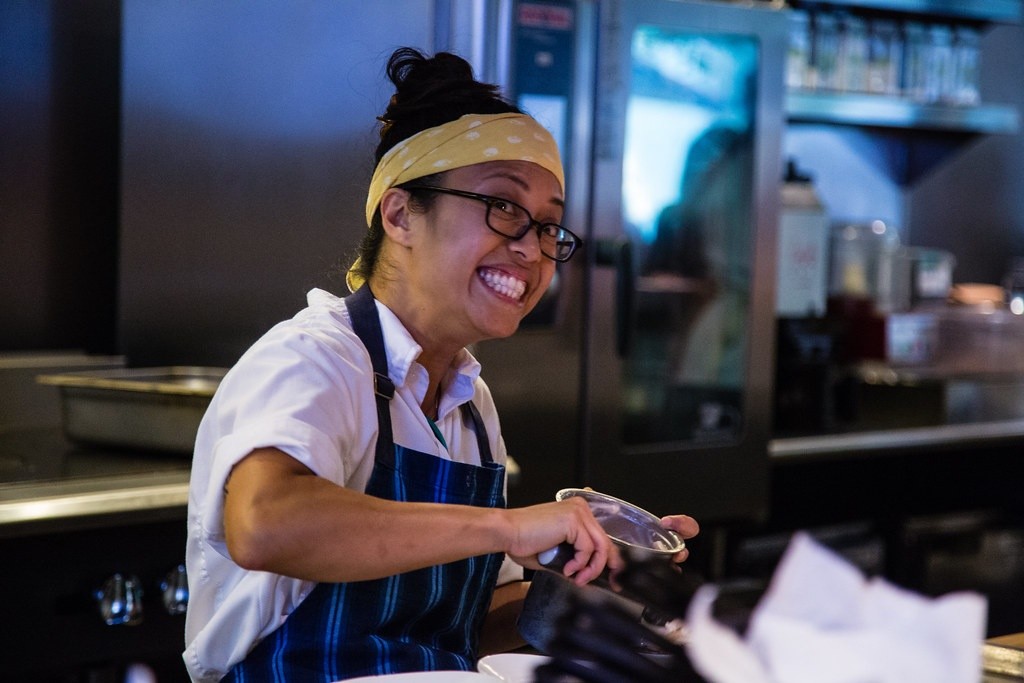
left=555, top=488, right=686, bottom=567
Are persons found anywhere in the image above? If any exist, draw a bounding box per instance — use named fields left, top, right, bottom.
left=182, top=44, right=703, bottom=683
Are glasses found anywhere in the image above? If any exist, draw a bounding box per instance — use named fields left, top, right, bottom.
left=423, top=183, right=584, bottom=263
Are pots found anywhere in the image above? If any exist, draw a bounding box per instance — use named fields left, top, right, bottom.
left=513, top=536, right=768, bottom=683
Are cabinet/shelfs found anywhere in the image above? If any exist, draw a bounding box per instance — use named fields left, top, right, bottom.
left=597, top=0, right=1024, bottom=639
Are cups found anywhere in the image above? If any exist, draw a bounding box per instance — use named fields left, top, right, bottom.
left=886, top=310, right=939, bottom=368
